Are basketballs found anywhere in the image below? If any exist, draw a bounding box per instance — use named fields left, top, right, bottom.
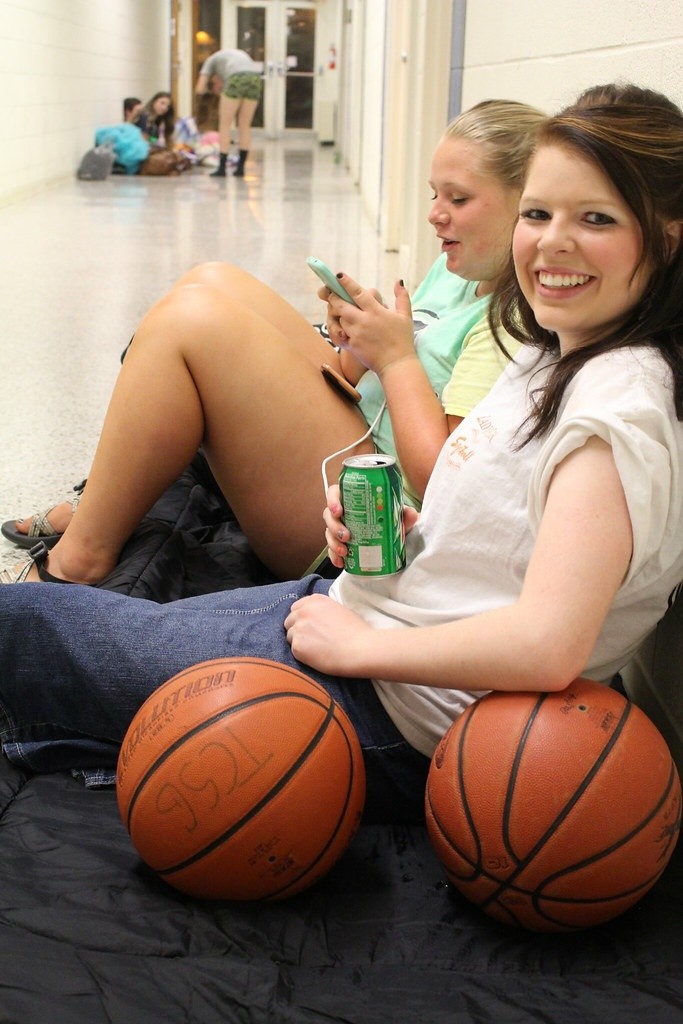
left=424, top=676, right=683, bottom=935
left=115, top=655, right=368, bottom=907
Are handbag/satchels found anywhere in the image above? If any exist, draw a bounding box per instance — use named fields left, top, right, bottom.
left=77, top=138, right=116, bottom=180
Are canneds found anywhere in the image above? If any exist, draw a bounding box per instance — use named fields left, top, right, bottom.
left=339, top=453, right=406, bottom=578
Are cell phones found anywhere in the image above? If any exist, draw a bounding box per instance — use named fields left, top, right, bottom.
left=321, top=364, right=362, bottom=405
left=307, top=255, right=359, bottom=308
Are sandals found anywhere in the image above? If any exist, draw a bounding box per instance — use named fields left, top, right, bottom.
left=2, top=496, right=82, bottom=546
left=0, top=541, right=98, bottom=587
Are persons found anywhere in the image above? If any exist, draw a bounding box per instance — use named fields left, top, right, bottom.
left=195, top=47, right=264, bottom=177
left=2, top=98, right=551, bottom=605
left=121, top=91, right=194, bottom=174
left=0, top=87, right=683, bottom=793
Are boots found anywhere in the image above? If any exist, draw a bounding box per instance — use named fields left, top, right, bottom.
left=233, top=149, right=248, bottom=177
left=209, top=153, right=227, bottom=177
